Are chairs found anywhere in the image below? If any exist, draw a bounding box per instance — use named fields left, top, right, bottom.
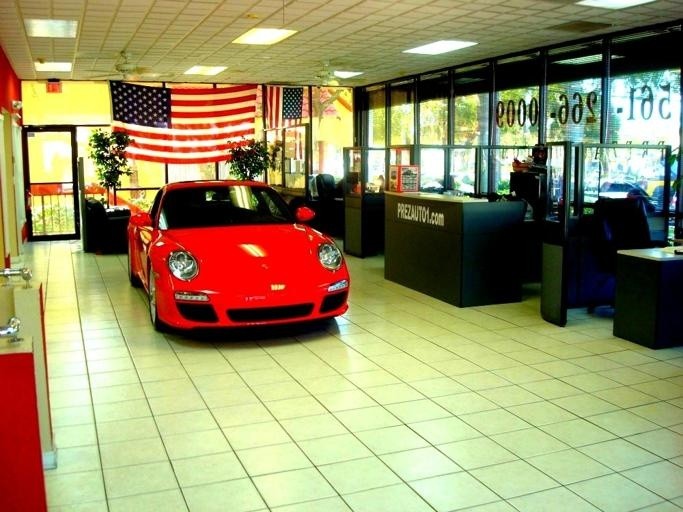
left=85, top=197, right=130, bottom=255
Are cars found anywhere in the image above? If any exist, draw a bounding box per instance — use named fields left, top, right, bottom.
left=599, top=179, right=675, bottom=212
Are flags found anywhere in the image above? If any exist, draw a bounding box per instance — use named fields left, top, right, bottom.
left=262, top=84, right=304, bottom=131
left=107, top=78, right=259, bottom=165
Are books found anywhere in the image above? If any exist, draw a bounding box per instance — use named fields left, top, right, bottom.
left=402, top=191, right=439, bottom=196
left=655, top=245, right=682, bottom=254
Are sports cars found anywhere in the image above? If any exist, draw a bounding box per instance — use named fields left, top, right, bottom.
left=127, top=178, right=349, bottom=332
